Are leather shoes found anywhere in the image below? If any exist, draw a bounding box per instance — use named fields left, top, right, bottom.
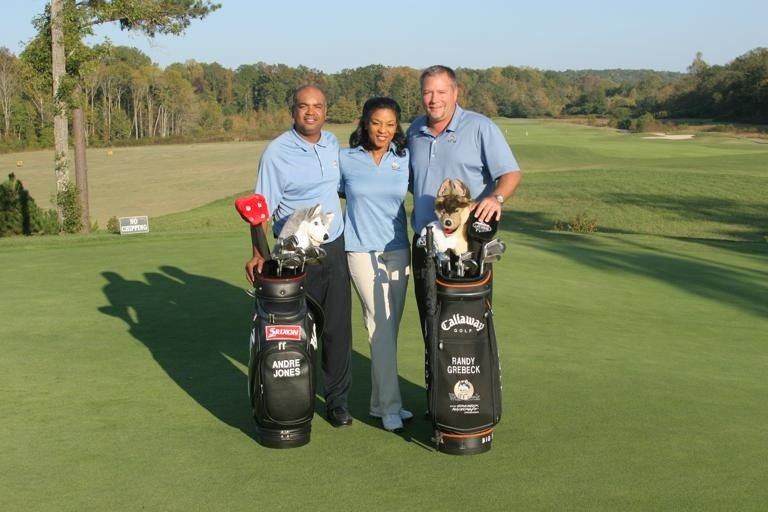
left=327, top=407, right=352, bottom=427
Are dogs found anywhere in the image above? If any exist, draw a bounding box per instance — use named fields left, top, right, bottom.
left=415, top=177, right=479, bottom=257
left=276, top=203, right=336, bottom=267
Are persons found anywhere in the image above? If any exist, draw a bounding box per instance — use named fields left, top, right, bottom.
left=335, top=97, right=414, bottom=433
left=405, top=65, right=522, bottom=418
left=245, top=85, right=353, bottom=428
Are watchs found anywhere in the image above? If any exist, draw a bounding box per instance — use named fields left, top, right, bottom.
left=493, top=193, right=505, bottom=204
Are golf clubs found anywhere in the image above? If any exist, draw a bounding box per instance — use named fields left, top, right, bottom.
left=270, top=236, right=327, bottom=277
left=417, top=221, right=505, bottom=278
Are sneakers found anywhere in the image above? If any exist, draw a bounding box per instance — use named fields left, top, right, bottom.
left=370, top=408, right=413, bottom=432
